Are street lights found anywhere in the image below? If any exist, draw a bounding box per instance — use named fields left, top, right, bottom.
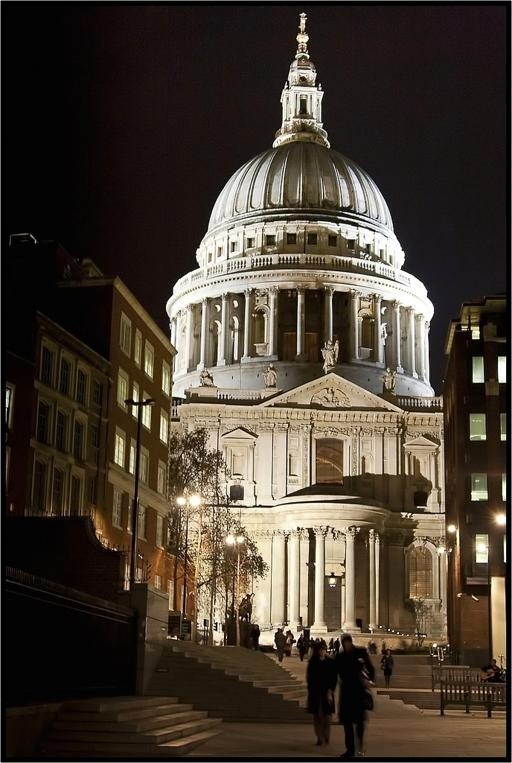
left=438, top=524, right=458, bottom=659
left=225, top=534, right=245, bottom=646
left=2, top=230, right=42, bottom=521
left=122, top=395, right=157, bottom=599
left=173, top=495, right=202, bottom=641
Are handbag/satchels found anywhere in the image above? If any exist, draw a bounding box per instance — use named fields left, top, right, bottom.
left=360, top=665, right=377, bottom=688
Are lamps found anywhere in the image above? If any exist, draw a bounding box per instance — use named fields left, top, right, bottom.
left=327, top=572, right=339, bottom=588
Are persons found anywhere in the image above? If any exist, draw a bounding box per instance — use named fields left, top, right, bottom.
left=380, top=367, right=396, bottom=392
left=479, top=658, right=503, bottom=682
left=249, top=622, right=393, bottom=757
left=264, top=364, right=278, bottom=387
left=320, top=339, right=341, bottom=372
